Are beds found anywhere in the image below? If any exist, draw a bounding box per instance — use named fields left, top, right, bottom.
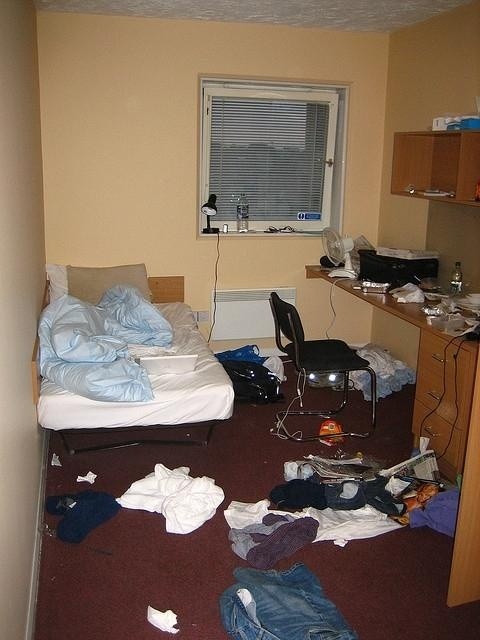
left=35, top=274, right=233, bottom=446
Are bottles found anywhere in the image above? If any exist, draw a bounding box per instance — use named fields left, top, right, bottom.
left=450, top=262, right=463, bottom=295
left=236, top=192, right=249, bottom=231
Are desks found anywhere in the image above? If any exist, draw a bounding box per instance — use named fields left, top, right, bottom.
left=305, top=265, right=480, bottom=607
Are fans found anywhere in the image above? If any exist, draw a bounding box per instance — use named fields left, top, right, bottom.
left=317, top=225, right=360, bottom=280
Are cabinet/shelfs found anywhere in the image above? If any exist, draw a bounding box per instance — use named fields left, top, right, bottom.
left=390, top=129, right=480, bottom=207
left=409, top=326, right=478, bottom=486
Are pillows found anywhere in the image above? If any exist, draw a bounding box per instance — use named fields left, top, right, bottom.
left=69, top=265, right=148, bottom=303
left=45, top=262, right=71, bottom=305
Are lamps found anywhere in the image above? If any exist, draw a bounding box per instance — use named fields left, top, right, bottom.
left=201, top=194, right=218, bottom=233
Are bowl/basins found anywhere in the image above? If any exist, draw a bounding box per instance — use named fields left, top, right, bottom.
left=361, top=283, right=389, bottom=293
left=466, top=294, right=480, bottom=305
left=431, top=318, right=465, bottom=329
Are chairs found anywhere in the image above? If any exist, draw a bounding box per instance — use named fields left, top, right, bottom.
left=268, top=291, right=377, bottom=442
